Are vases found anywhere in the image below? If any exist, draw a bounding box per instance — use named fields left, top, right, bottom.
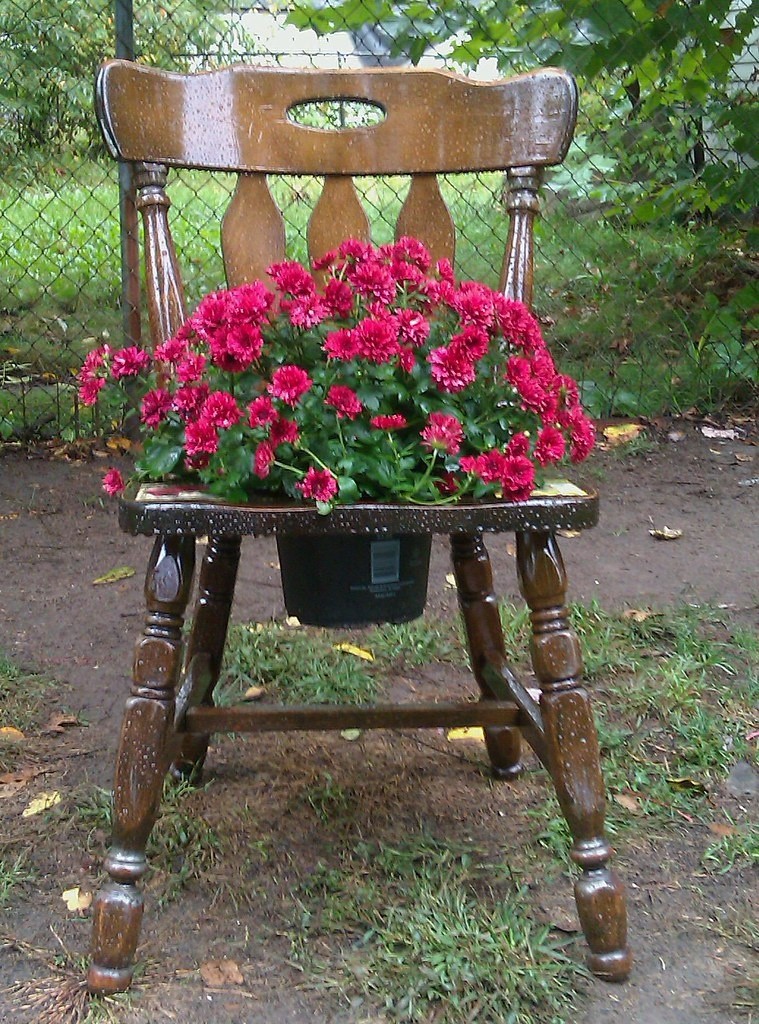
left=271, top=531, right=435, bottom=630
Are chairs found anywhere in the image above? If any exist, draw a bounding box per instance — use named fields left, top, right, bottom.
left=81, top=59, right=636, bottom=999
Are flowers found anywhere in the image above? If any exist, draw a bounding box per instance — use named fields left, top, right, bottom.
left=75, top=233, right=598, bottom=516
